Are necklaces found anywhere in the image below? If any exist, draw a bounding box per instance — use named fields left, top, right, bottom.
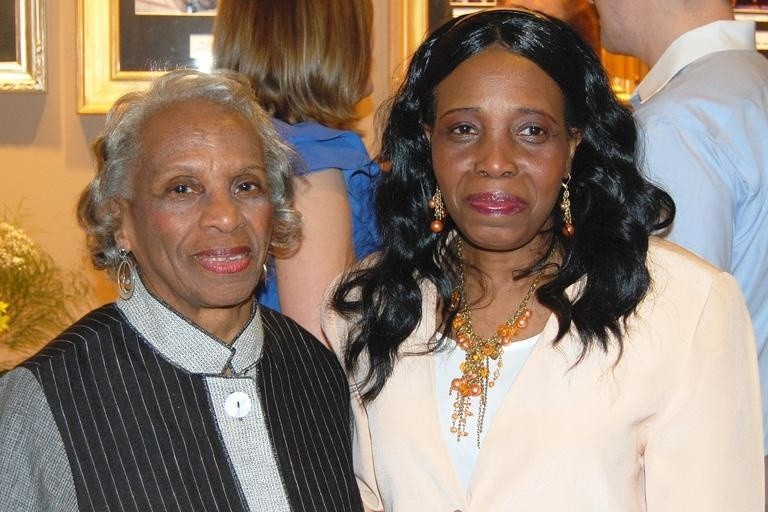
left=449, top=232, right=558, bottom=450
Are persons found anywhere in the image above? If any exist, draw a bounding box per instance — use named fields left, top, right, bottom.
left=211, top=0, right=390, bottom=358
left=591, top=0, right=764, bottom=458
left=0, top=67, right=364, bottom=511
left=320, top=6, right=764, bottom=512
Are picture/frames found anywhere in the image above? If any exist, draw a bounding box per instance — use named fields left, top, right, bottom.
left=1, top=0, right=222, bottom=113
